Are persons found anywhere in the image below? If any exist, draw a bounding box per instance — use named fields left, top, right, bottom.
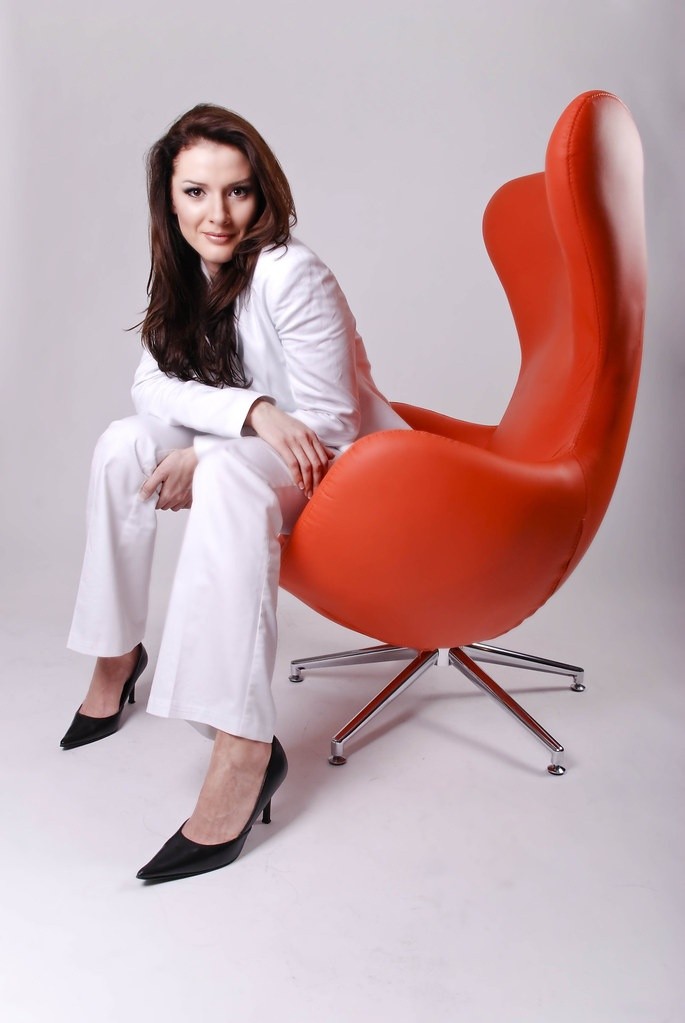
left=59, top=104, right=411, bottom=879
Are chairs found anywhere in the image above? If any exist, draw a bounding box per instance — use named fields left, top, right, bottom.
left=276, top=93, right=649, bottom=775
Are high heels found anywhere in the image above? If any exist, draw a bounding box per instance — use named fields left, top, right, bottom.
left=137, top=735, right=289, bottom=880
left=60, top=641, right=150, bottom=751
